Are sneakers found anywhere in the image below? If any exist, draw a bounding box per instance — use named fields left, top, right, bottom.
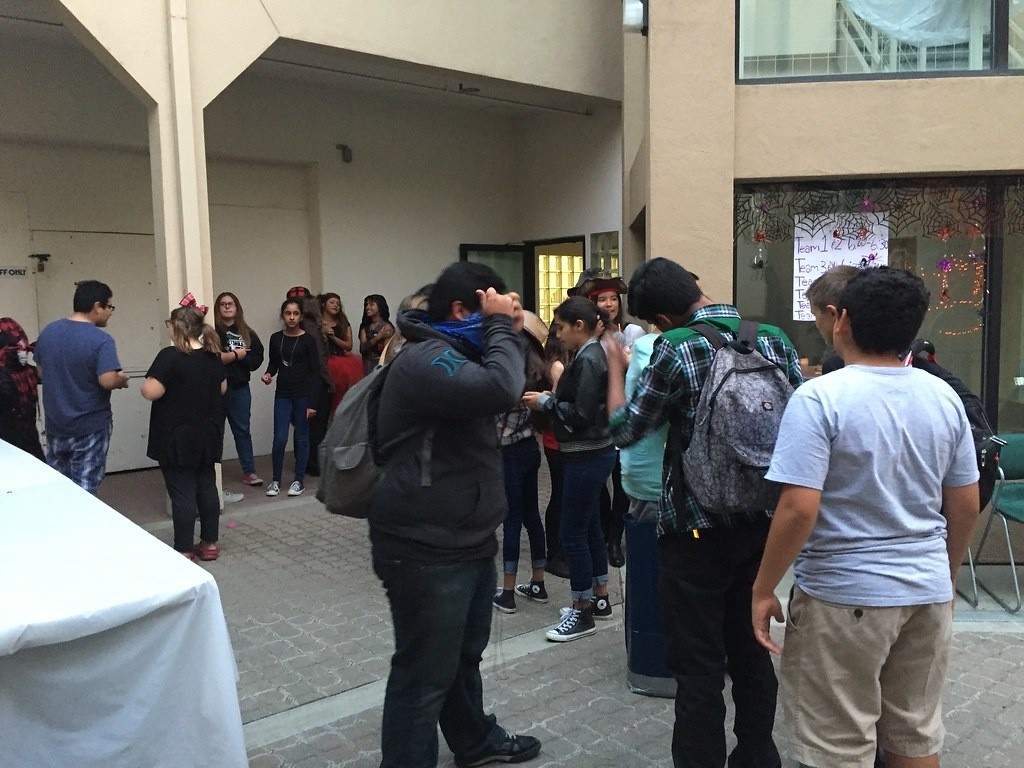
left=493, top=586, right=516, bottom=613
left=545, top=598, right=598, bottom=641
left=559, top=592, right=613, bottom=620
left=515, top=576, right=548, bottom=603
left=455, top=731, right=542, bottom=768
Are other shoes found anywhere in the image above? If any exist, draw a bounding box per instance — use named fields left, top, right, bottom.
left=286, top=480, right=305, bottom=495
left=194, top=542, right=219, bottom=560
left=265, top=481, right=281, bottom=496
left=243, top=474, right=263, bottom=485
left=182, top=552, right=198, bottom=564
left=222, top=491, right=244, bottom=503
left=609, top=535, right=625, bottom=568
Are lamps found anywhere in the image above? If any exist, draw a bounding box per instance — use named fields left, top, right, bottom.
left=620, top=0, right=648, bottom=35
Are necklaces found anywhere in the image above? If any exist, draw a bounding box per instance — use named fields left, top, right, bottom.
left=279, top=330, right=300, bottom=367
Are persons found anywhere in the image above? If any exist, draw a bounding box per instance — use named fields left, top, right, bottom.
left=1, top=317, right=45, bottom=463
left=140, top=306, right=252, bottom=564
left=263, top=255, right=1004, bottom=768
left=213, top=295, right=265, bottom=489
left=30, top=279, right=129, bottom=497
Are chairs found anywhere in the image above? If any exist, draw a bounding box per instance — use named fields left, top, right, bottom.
left=974, top=433, right=1024, bottom=613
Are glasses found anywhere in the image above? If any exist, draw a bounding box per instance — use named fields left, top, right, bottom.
left=219, top=300, right=235, bottom=307
left=164, top=319, right=172, bottom=327
left=100, top=302, right=115, bottom=312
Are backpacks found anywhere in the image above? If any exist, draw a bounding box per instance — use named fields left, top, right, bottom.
left=909, top=353, right=1008, bottom=514
left=674, top=315, right=799, bottom=515
left=314, top=339, right=421, bottom=517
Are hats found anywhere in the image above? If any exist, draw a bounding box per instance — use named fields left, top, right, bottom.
left=286, top=286, right=315, bottom=303
left=567, top=267, right=628, bottom=297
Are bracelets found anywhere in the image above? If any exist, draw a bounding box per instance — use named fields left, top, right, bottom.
left=233, top=350, right=239, bottom=360
